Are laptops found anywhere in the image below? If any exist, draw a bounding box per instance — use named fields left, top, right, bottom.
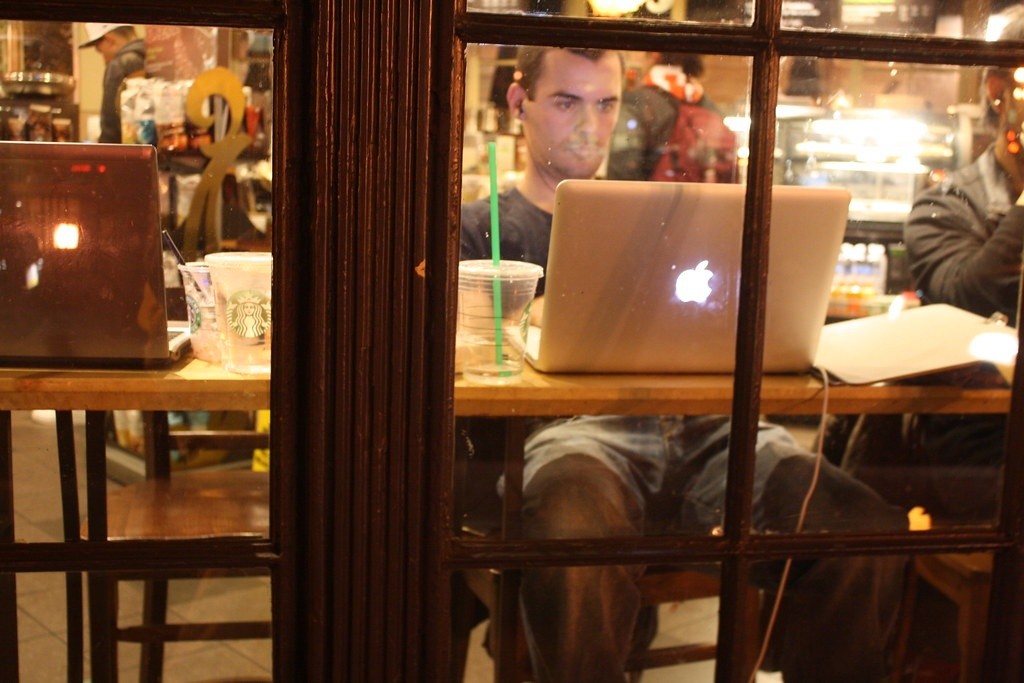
left=505, top=178, right=851, bottom=374
left=0, top=140, right=191, bottom=365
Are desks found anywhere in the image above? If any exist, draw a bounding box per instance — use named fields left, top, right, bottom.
left=0, top=317, right=1022, bottom=683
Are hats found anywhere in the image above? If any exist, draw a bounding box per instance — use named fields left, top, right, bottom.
left=77, top=23, right=133, bottom=49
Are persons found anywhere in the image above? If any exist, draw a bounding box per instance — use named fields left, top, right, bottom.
left=906, top=13, right=1024, bottom=338
left=74, top=23, right=151, bottom=151
left=454, top=38, right=915, bottom=682
left=606, top=39, right=745, bottom=191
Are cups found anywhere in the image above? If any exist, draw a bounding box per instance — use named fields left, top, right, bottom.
left=176, top=261, right=223, bottom=362
left=459, top=259, right=545, bottom=390
left=204, top=251, right=272, bottom=376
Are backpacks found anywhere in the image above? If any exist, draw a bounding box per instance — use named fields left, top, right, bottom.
left=651, top=103, right=739, bottom=184
